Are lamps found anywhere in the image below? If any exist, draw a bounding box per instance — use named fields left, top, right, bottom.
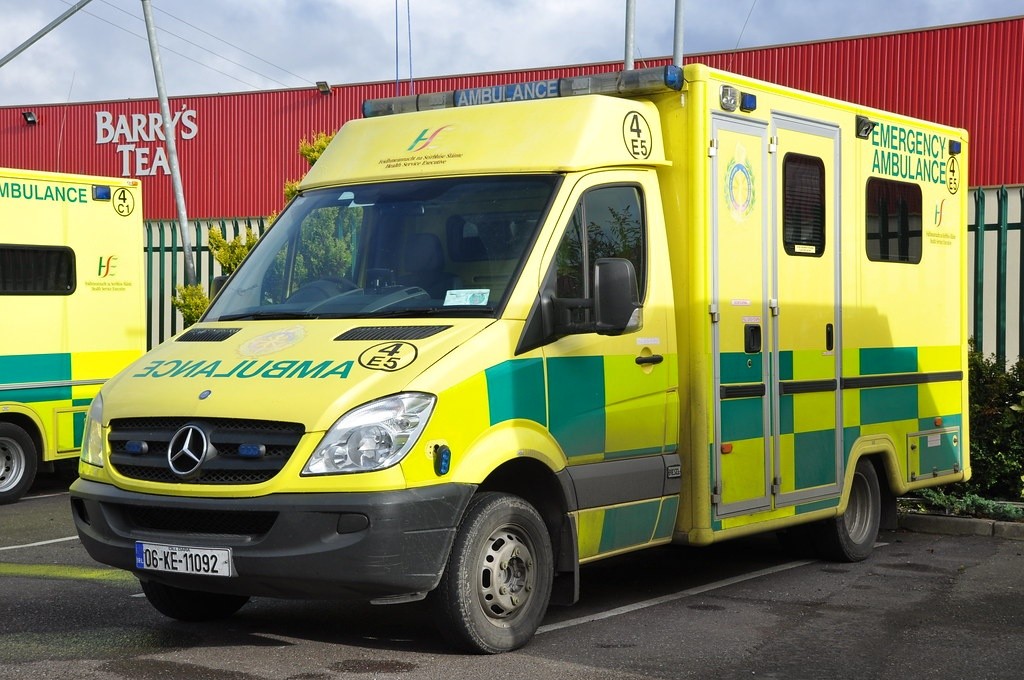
left=22, top=111, right=38, bottom=124
left=316, top=81, right=333, bottom=94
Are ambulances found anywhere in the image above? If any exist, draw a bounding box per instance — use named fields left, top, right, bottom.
left=69, top=63, right=972, bottom=655
left=0, top=166, right=148, bottom=505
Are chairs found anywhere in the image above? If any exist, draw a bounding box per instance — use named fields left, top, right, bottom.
left=396, top=231, right=464, bottom=301
